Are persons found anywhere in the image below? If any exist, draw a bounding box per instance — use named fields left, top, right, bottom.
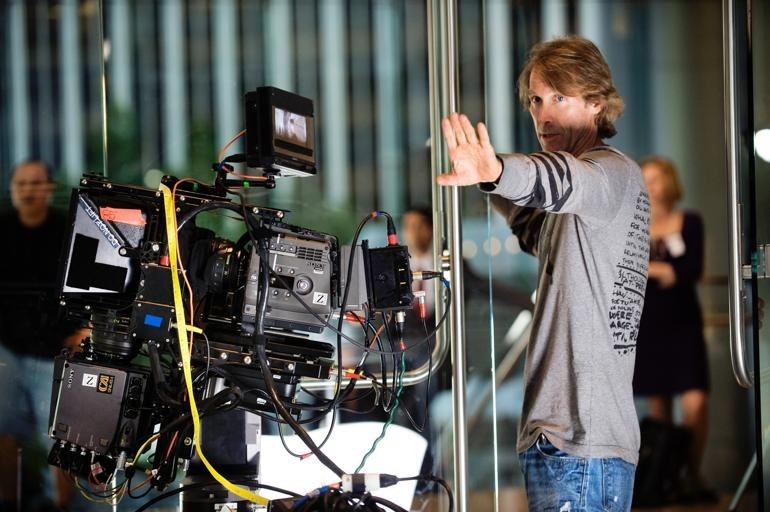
left=436, top=36, right=651, bottom=511
left=638, top=155, right=723, bottom=506
left=0, top=160, right=100, bottom=512
left=357, top=202, right=540, bottom=495
